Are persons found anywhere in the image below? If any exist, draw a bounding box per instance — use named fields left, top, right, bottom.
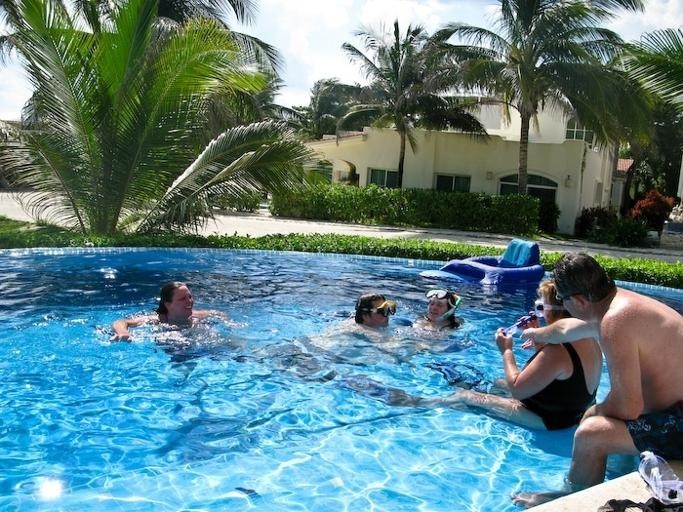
left=267, top=292, right=422, bottom=408
left=110, top=280, right=246, bottom=365
left=432, top=274, right=604, bottom=434
left=402, top=287, right=477, bottom=355
left=507, top=252, right=682, bottom=509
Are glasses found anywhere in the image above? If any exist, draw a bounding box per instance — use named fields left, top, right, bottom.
left=425, top=289, right=451, bottom=299
left=556, top=293, right=582, bottom=304
left=355, top=300, right=396, bottom=317
left=535, top=299, right=567, bottom=317
